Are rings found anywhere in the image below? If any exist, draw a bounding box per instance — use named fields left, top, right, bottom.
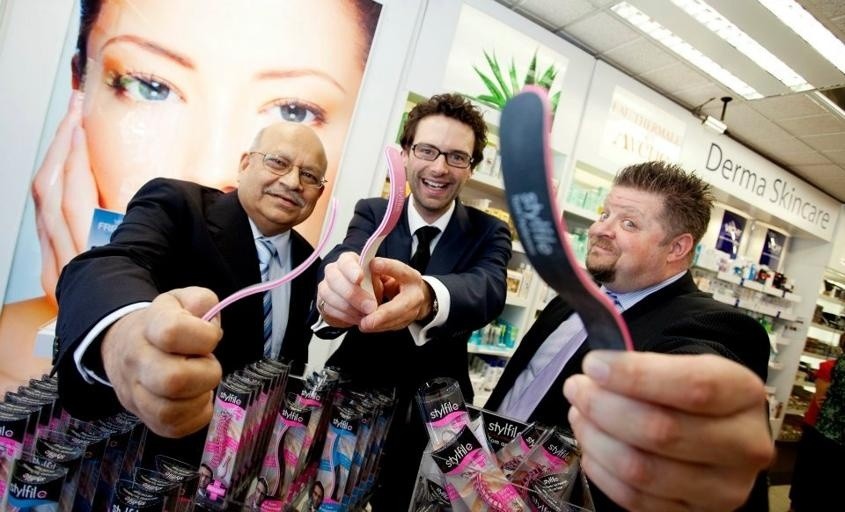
left=318, top=300, right=325, bottom=312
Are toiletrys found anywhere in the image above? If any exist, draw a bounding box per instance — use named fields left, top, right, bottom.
left=467, top=260, right=534, bottom=391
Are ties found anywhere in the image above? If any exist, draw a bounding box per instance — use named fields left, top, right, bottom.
left=410, top=227, right=440, bottom=275
left=254, top=238, right=278, bottom=358
left=499, top=293, right=622, bottom=423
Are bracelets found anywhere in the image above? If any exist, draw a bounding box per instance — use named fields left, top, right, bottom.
left=433, top=298, right=438, bottom=320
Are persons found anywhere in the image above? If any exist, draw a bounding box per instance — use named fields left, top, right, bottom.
left=50, top=123, right=328, bottom=441
left=311, top=93, right=514, bottom=512
left=198, top=464, right=213, bottom=497
left=789, top=331, right=845, bottom=512
left=0, top=0, right=383, bottom=408
left=310, top=480, right=325, bottom=509
left=480, top=161, right=779, bottom=511
left=246, top=477, right=269, bottom=507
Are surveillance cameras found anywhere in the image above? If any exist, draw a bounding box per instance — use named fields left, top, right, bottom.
left=701, top=114, right=728, bottom=134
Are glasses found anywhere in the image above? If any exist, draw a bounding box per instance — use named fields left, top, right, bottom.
left=249, top=151, right=328, bottom=189
left=408, top=144, right=475, bottom=168
